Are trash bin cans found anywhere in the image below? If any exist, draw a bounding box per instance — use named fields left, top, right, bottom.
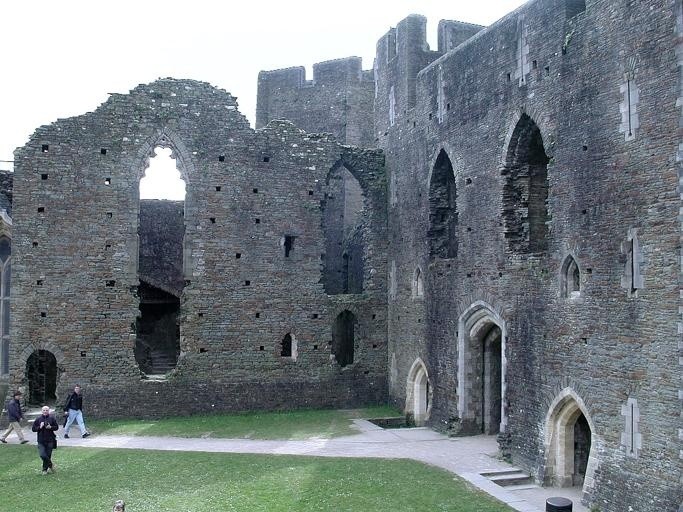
left=546, top=497, right=573, bottom=512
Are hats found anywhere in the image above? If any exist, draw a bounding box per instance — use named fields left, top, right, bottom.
left=11, top=390, right=23, bottom=397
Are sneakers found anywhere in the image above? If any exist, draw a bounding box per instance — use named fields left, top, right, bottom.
left=0, top=438, right=7, bottom=443
left=21, top=440, right=28, bottom=444
left=43, top=467, right=55, bottom=474
left=64, top=432, right=90, bottom=438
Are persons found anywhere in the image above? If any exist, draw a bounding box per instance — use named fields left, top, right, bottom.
left=62, top=383, right=89, bottom=438
left=0, top=390, right=28, bottom=444
left=31, top=406, right=58, bottom=475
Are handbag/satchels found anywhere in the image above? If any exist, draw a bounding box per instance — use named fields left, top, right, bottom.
left=53, top=439, right=58, bottom=449
